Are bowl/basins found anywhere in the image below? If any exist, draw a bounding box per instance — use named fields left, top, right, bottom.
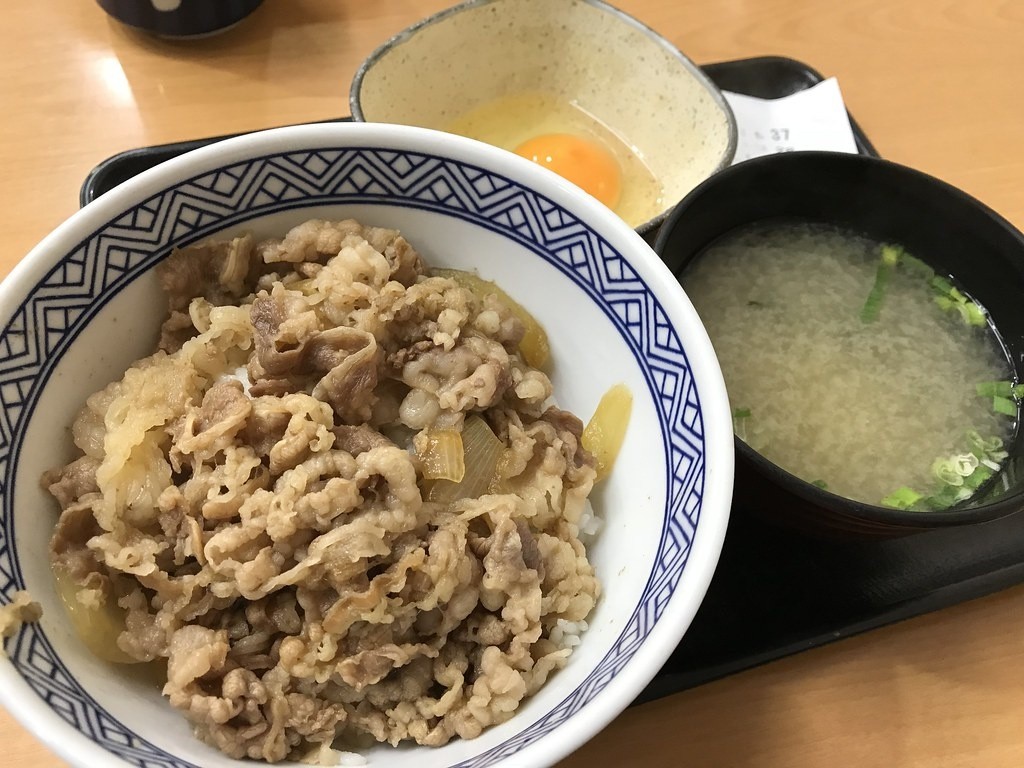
left=348, top=0, right=735, bottom=238
left=94, top=0, right=265, bottom=41
left=0, top=121, right=740, bottom=768
left=649, top=150, right=1024, bottom=534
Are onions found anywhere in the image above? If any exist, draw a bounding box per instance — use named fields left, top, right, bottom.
left=52, top=270, right=638, bottom=666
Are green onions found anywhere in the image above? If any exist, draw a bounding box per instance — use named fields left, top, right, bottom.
left=733, top=238, right=1024, bottom=513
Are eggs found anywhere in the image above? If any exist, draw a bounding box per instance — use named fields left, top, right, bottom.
left=448, top=88, right=660, bottom=233
